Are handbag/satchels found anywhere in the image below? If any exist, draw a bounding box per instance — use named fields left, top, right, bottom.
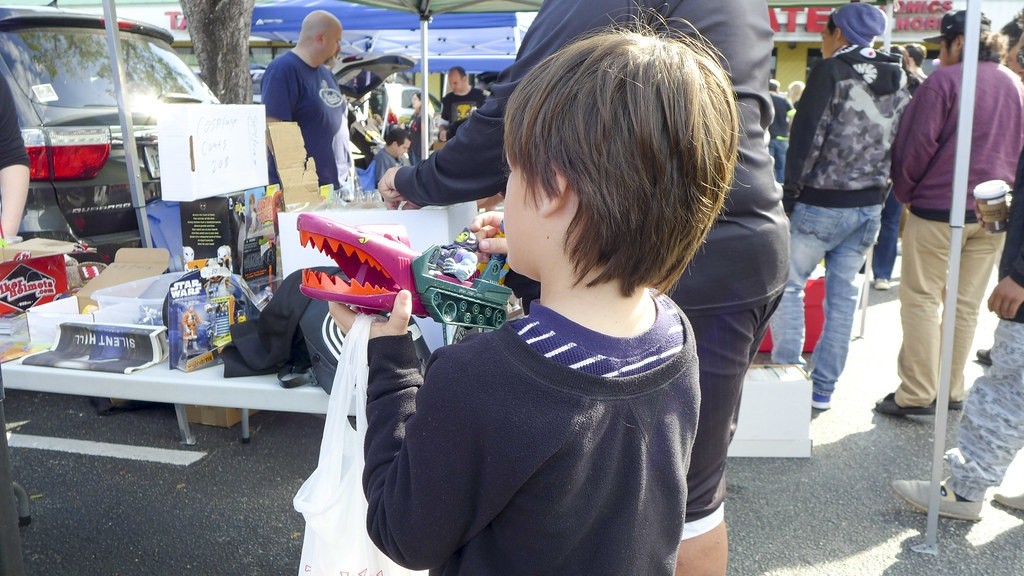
left=293, top=316, right=430, bottom=576
left=277, top=299, right=347, bottom=394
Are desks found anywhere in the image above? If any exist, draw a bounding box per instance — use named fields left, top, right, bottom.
left=0, top=313, right=332, bottom=444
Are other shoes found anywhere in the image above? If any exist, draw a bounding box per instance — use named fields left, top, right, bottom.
left=811, top=392, right=831, bottom=409
left=949, top=401, right=964, bottom=409
left=976, top=348, right=992, bottom=365
left=875, top=393, right=936, bottom=421
left=994, top=494, right=1024, bottom=510
left=893, top=476, right=982, bottom=520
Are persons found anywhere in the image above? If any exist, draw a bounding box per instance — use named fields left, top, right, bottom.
left=182, top=304, right=210, bottom=353
left=873, top=10, right=1024, bottom=415
left=260, top=9, right=355, bottom=203
left=0, top=74, right=31, bottom=238
left=373, top=129, right=412, bottom=189
left=438, top=227, right=479, bottom=282
left=438, top=65, right=498, bottom=142
left=769, top=4, right=913, bottom=410
left=377, top=0, right=791, bottom=575
left=890, top=27, right=1024, bottom=521
left=328, top=34, right=739, bottom=575
left=407, top=91, right=434, bottom=164
left=860, top=44, right=929, bottom=290
left=246, top=194, right=258, bottom=234
left=768, top=79, right=806, bottom=185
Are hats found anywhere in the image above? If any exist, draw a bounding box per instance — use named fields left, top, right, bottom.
left=923, top=9, right=989, bottom=45
left=832, top=2, right=886, bottom=48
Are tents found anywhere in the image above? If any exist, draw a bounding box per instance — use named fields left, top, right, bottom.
left=247, top=0, right=538, bottom=75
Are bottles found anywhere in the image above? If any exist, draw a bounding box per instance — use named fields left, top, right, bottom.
left=325, top=186, right=383, bottom=211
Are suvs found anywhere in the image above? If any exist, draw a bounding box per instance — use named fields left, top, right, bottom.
left=248, top=52, right=443, bottom=168
left=0, top=0, right=221, bottom=268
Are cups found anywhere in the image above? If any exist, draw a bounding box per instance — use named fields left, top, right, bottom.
left=972, top=179, right=1010, bottom=234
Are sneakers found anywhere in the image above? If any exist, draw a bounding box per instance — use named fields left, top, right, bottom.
left=874, top=278, right=890, bottom=290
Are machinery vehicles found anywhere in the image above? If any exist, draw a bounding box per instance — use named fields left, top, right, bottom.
left=412, top=242, right=514, bottom=331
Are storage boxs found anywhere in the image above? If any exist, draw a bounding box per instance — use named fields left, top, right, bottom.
left=751, top=268, right=856, bottom=354
left=727, top=362, right=820, bottom=459
left=186, top=404, right=258, bottom=427
left=0, top=98, right=479, bottom=428
